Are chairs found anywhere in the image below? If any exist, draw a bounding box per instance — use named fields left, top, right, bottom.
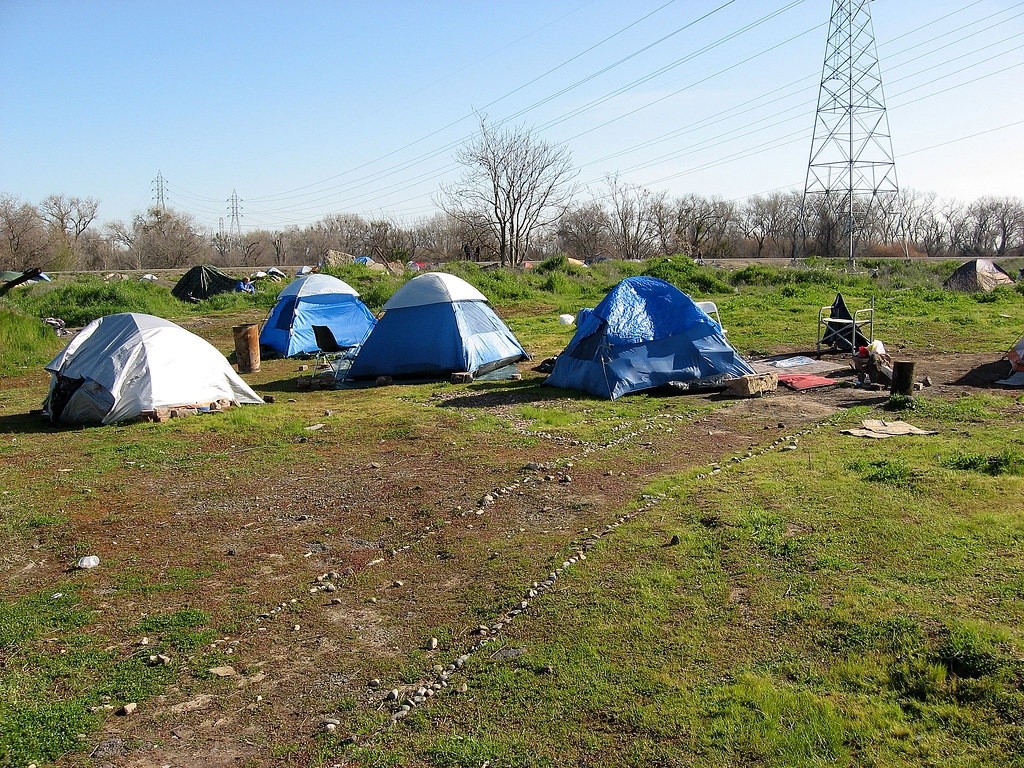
left=310, top=324, right=358, bottom=382
left=693, top=301, right=728, bottom=336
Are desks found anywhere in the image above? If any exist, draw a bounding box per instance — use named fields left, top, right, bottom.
left=814, top=305, right=876, bottom=362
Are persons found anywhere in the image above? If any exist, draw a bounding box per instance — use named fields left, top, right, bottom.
left=235, top=277, right=255, bottom=295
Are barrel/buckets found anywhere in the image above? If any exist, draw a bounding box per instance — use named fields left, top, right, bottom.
left=232, top=323, right=261, bottom=373
left=853, top=351, right=870, bottom=371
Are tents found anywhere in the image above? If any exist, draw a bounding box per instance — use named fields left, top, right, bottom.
left=170, top=255, right=432, bottom=305
left=542, top=276, right=759, bottom=402
left=942, top=257, right=1016, bottom=295
left=334, top=272, right=531, bottom=381
left=42, top=312, right=267, bottom=425
left=258, top=273, right=378, bottom=359
